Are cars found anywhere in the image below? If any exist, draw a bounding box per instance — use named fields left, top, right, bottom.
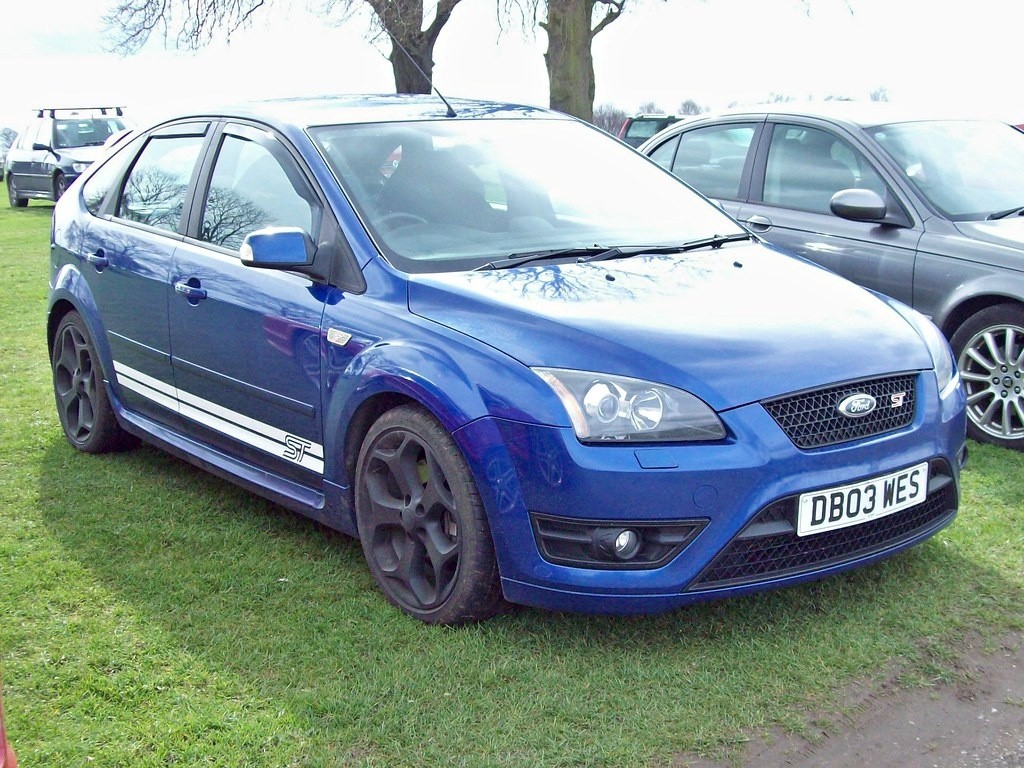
left=46, top=93, right=969, bottom=627
left=629, top=101, right=1024, bottom=451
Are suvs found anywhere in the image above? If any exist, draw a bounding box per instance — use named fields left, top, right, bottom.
left=616, top=113, right=690, bottom=148
left=4, top=108, right=130, bottom=207
